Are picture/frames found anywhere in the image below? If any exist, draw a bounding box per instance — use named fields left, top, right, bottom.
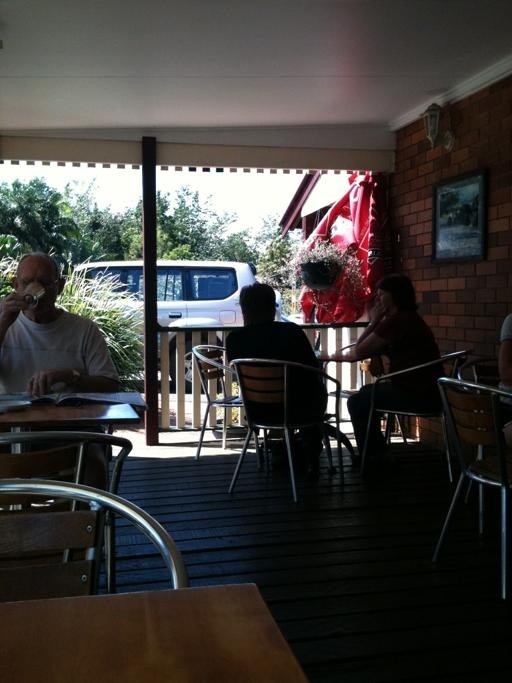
left=430, top=166, right=487, bottom=264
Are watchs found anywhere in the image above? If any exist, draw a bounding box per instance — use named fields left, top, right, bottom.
left=72, top=369, right=81, bottom=385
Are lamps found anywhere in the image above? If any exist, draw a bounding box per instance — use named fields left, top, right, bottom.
left=419, top=102, right=456, bottom=152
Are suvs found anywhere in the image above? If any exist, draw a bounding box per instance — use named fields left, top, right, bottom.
left=70, top=260, right=282, bottom=393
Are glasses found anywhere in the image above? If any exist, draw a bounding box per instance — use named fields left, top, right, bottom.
left=16, top=276, right=59, bottom=289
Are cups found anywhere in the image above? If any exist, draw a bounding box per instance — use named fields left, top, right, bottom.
left=19, top=280, right=44, bottom=314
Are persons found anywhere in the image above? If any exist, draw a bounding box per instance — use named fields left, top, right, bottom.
left=498, top=311, right=512, bottom=387
left=0, top=255, right=119, bottom=494
left=227, top=283, right=328, bottom=476
left=347, top=273, right=444, bottom=469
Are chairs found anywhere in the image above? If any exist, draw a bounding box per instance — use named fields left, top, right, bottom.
left=323, top=341, right=379, bottom=449
left=227, top=357, right=345, bottom=501
left=456, top=363, right=487, bottom=534
left=1, top=474, right=186, bottom=598
left=358, top=348, right=470, bottom=504
left=191, top=343, right=263, bottom=469
left=430, top=376, right=512, bottom=597
left=0, top=430, right=134, bottom=593
